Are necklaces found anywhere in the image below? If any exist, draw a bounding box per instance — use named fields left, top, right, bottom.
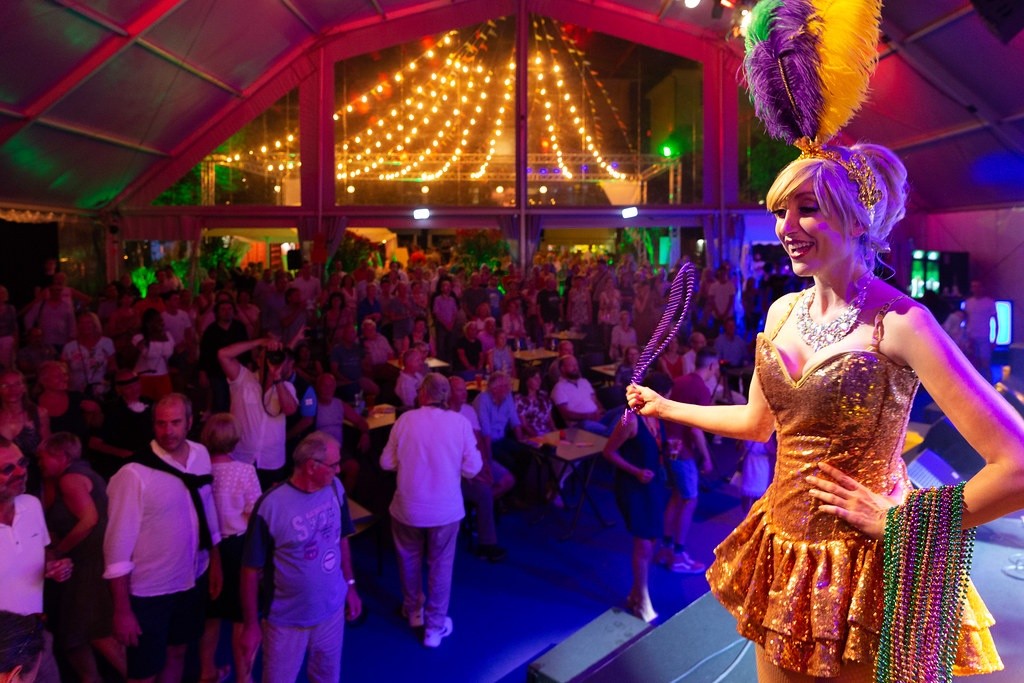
left=872, top=481, right=976, bottom=683
left=621, top=262, right=694, bottom=424
left=795, top=272, right=875, bottom=353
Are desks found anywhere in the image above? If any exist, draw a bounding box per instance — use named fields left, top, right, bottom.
left=335, top=326, right=672, bottom=584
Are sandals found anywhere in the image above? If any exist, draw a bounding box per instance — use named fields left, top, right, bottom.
left=201, top=665, right=232, bottom=683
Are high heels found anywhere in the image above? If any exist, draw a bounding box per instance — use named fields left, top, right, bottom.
left=629, top=594, right=658, bottom=624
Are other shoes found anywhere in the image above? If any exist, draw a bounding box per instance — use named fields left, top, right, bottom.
left=477, top=542, right=507, bottom=562
left=504, top=500, right=530, bottom=516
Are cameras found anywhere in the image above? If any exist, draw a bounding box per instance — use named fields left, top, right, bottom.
left=266, top=348, right=286, bottom=366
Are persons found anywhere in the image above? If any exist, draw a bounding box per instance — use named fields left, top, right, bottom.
left=0, top=249, right=1024, bottom=683
left=625, top=145, right=1024, bottom=683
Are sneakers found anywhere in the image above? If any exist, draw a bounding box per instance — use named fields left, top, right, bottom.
left=655, top=548, right=671, bottom=564
left=668, top=553, right=705, bottom=572
left=403, top=605, right=424, bottom=626
left=423, top=616, right=453, bottom=647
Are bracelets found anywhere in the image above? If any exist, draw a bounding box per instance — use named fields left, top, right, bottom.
left=348, top=579, right=355, bottom=585
left=273, top=379, right=282, bottom=384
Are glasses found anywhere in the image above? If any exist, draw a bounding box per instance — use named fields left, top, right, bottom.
left=1, top=382, right=19, bottom=389
left=0, top=457, right=29, bottom=474
left=315, top=458, right=339, bottom=469
